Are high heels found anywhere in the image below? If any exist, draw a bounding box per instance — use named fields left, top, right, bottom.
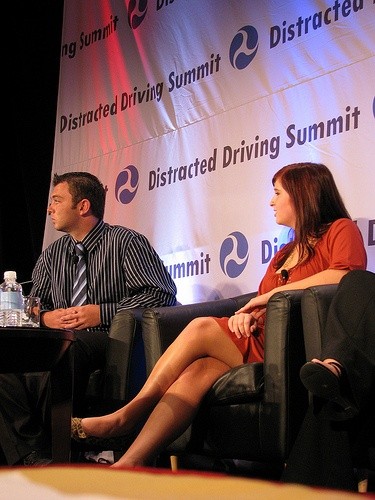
left=299, top=362, right=359, bottom=417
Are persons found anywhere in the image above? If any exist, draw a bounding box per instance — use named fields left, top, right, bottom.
left=68, top=163, right=369, bottom=469
left=273, top=269, right=375, bottom=488
left=0, top=172, right=178, bottom=466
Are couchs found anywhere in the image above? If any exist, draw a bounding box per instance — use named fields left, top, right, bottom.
left=21, top=308, right=146, bottom=463
left=143, top=289, right=306, bottom=482
left=301, top=283, right=375, bottom=495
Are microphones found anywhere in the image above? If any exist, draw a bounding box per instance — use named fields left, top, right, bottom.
left=72, top=256, right=79, bottom=264
left=281, top=269, right=288, bottom=280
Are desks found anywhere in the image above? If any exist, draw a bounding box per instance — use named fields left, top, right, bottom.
left=0, top=327, right=84, bottom=464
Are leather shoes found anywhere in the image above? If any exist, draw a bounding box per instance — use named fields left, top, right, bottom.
left=72, top=418, right=136, bottom=450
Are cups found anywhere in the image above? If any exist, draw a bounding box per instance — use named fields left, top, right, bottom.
left=23, top=296, right=41, bottom=327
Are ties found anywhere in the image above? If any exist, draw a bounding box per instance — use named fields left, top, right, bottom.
left=70, top=244, right=87, bottom=306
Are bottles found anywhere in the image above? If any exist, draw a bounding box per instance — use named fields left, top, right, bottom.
left=0, top=271, right=23, bottom=327
